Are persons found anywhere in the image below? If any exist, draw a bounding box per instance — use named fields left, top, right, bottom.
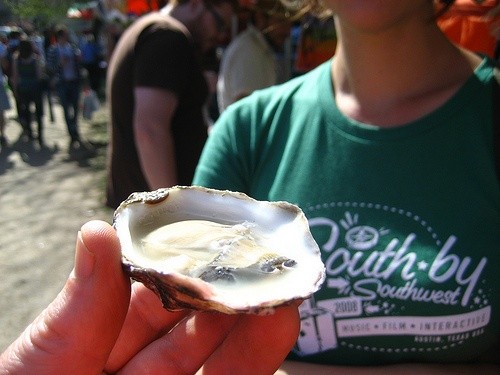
left=106, top=0, right=225, bottom=209
left=215, top=0, right=291, bottom=115
left=0, top=215, right=303, bottom=375
left=48, top=29, right=82, bottom=147
left=0, top=24, right=47, bottom=149
left=295, top=1, right=338, bottom=76
left=79, top=17, right=120, bottom=88
left=189, top=0, right=500, bottom=374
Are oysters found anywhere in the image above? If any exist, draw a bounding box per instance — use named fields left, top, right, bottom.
left=107, top=184, right=327, bottom=313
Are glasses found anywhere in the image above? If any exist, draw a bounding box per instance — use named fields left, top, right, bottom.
left=206, top=2, right=234, bottom=35
